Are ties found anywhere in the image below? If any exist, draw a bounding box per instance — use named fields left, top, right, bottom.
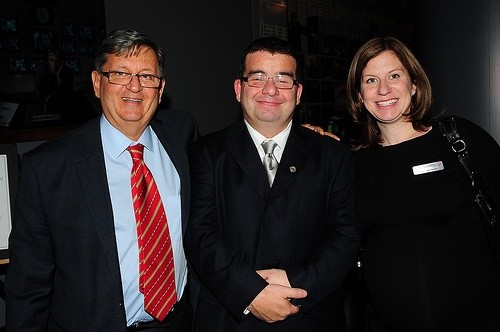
left=126, top=143, right=178, bottom=320
left=261, top=141, right=278, bottom=188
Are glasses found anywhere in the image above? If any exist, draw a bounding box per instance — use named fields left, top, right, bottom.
left=242, top=73, right=298, bottom=89
left=99, top=70, right=161, bottom=89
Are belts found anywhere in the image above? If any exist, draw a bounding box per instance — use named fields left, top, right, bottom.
left=127, top=298, right=191, bottom=332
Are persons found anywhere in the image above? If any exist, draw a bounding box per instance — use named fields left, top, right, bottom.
left=0, top=26, right=192, bottom=331
left=183, top=37, right=359, bottom=332
left=43, top=42, right=73, bottom=91
left=302, top=37, right=500, bottom=332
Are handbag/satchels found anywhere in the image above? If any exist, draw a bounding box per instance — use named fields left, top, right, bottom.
left=438, top=115, right=500, bottom=236
